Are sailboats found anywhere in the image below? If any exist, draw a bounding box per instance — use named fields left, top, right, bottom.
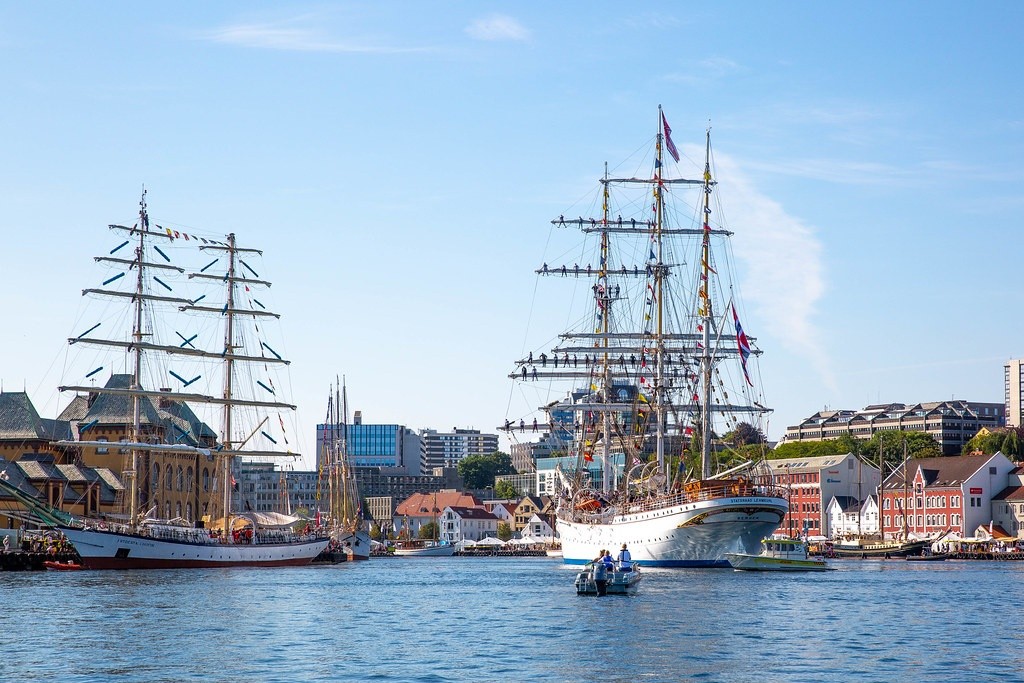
left=0, top=182, right=335, bottom=571
left=493, top=103, right=796, bottom=571
left=310, top=373, right=372, bottom=561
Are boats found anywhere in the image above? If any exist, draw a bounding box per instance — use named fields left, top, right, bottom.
left=905, top=552, right=949, bottom=561
left=43, top=560, right=82, bottom=571
left=825, top=434, right=952, bottom=561
left=393, top=540, right=455, bottom=557
left=722, top=549, right=827, bottom=572
left=574, top=496, right=602, bottom=511
left=574, top=558, right=643, bottom=596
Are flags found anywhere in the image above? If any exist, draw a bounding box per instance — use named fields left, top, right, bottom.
left=666, top=132, right=680, bottom=163
left=685, top=426, right=696, bottom=438
left=701, top=273, right=709, bottom=282
left=662, top=112, right=672, bottom=135
left=704, top=222, right=711, bottom=231
left=700, top=290, right=709, bottom=299
left=703, top=239, right=708, bottom=248
left=732, top=304, right=753, bottom=386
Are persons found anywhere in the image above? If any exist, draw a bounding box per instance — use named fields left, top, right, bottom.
left=520, top=419, right=525, bottom=433
left=541, top=262, right=667, bottom=299
left=549, top=418, right=579, bottom=433
left=539, top=352, right=597, bottom=369
left=532, top=418, right=538, bottom=432
left=618, top=544, right=632, bottom=571
left=598, top=550, right=616, bottom=571
left=3, top=535, right=9, bottom=551
left=558, top=214, right=651, bottom=228
left=618, top=354, right=688, bottom=381
left=505, top=419, right=515, bottom=432
left=209, top=528, right=252, bottom=545
left=522, top=351, right=538, bottom=381
left=596, top=548, right=606, bottom=562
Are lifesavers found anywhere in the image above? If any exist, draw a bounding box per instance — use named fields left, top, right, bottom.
left=932, top=551, right=1024, bottom=560
left=827, top=549, right=833, bottom=557
left=459, top=550, right=545, bottom=556
left=0, top=551, right=82, bottom=568
left=99, top=522, right=106, bottom=529
